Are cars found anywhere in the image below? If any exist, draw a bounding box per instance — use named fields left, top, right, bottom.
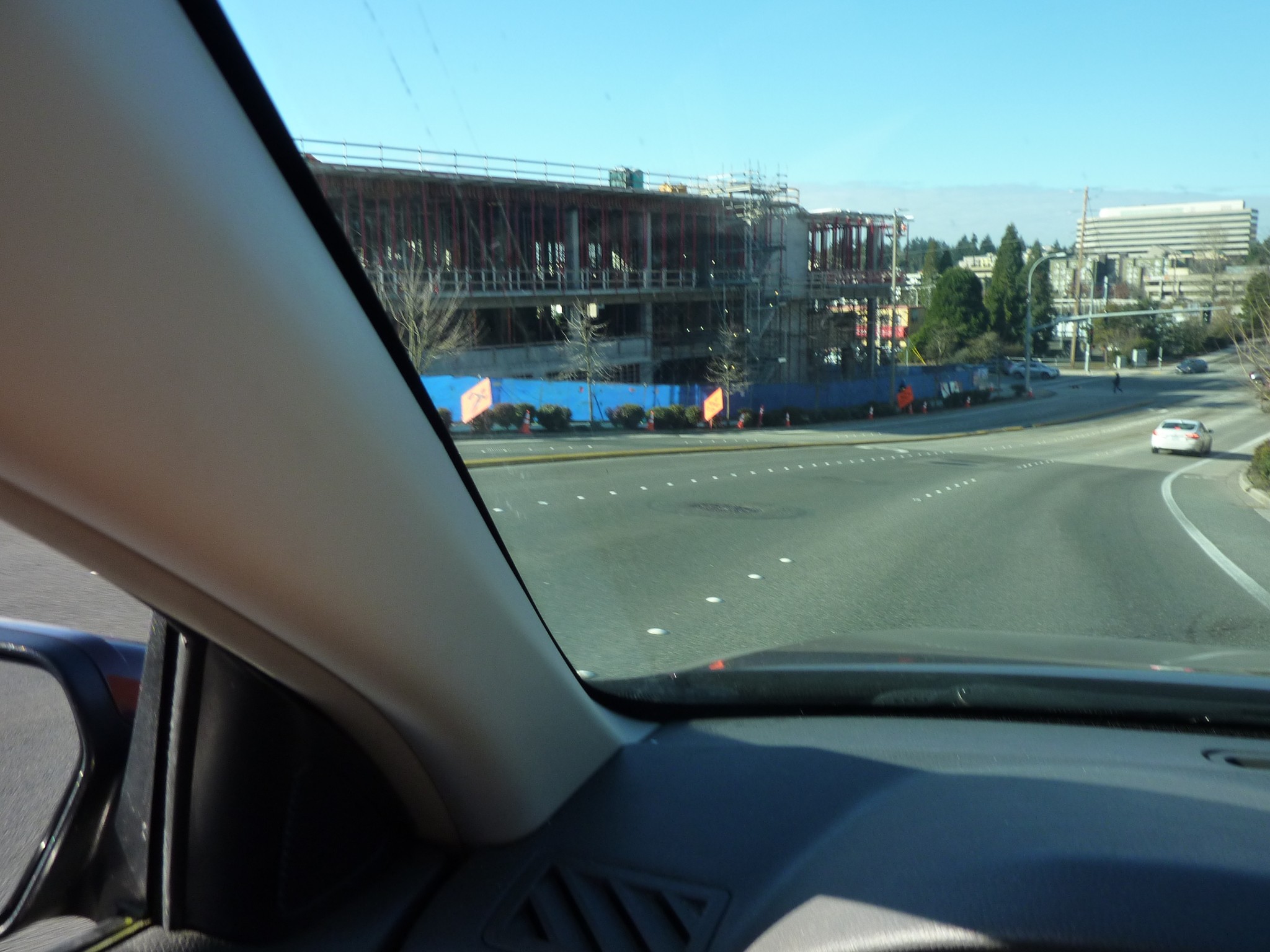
left=1175, top=357, right=1208, bottom=375
left=1151, top=418, right=1213, bottom=457
left=1008, top=360, right=1060, bottom=380
left=1247, top=367, right=1270, bottom=385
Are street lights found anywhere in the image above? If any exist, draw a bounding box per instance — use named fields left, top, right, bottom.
left=871, top=209, right=915, bottom=400
left=1024, top=252, right=1068, bottom=400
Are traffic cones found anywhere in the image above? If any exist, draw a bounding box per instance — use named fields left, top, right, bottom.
left=1028, top=386, right=1034, bottom=400
left=965, top=395, right=972, bottom=408
left=785, top=412, right=792, bottom=428
left=867, top=406, right=875, bottom=420
left=908, top=403, right=914, bottom=415
left=645, top=410, right=655, bottom=432
left=521, top=408, right=534, bottom=435
left=922, top=400, right=928, bottom=415
left=736, top=412, right=746, bottom=429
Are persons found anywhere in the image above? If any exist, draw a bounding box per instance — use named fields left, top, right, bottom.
left=1113, top=373, right=1123, bottom=393
left=899, top=379, right=906, bottom=392
